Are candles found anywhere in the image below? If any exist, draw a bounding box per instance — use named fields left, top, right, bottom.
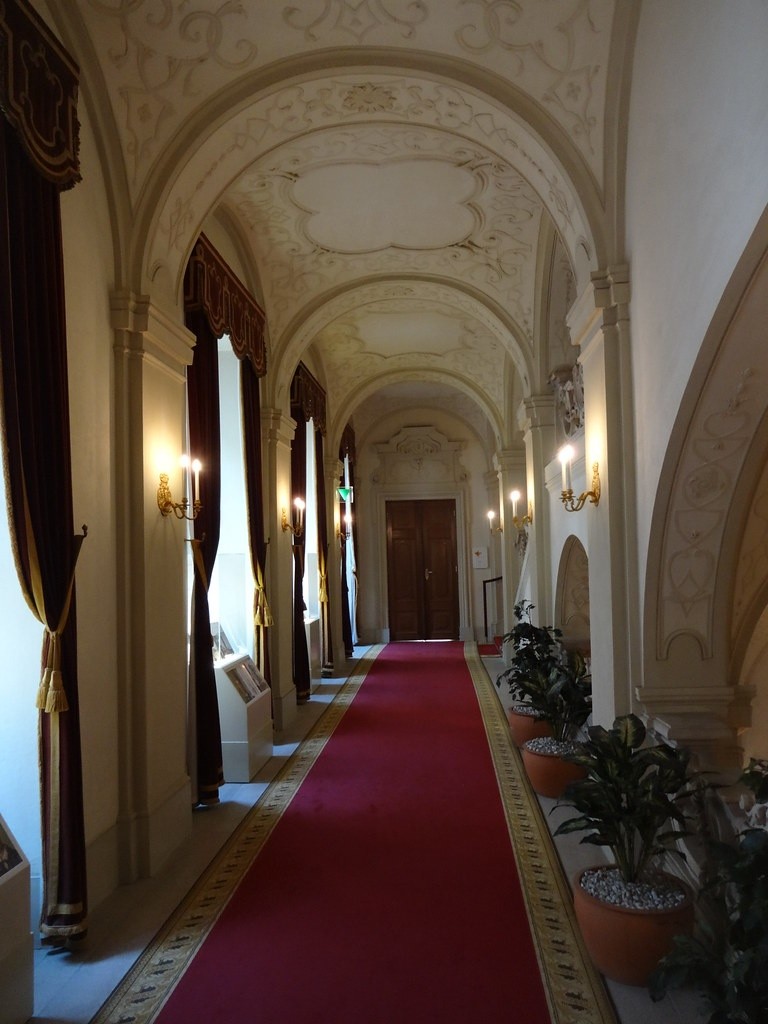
left=344, top=514, right=351, bottom=533
left=191, top=459, right=202, bottom=499
left=488, top=511, right=494, bottom=529
left=294, top=497, right=305, bottom=526
left=510, top=490, right=520, bottom=517
left=558, top=445, right=574, bottom=490
left=180, top=454, right=189, bottom=498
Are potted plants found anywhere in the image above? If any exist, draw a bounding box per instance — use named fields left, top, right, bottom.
left=496, top=600, right=592, bottom=799
left=547, top=710, right=730, bottom=988
